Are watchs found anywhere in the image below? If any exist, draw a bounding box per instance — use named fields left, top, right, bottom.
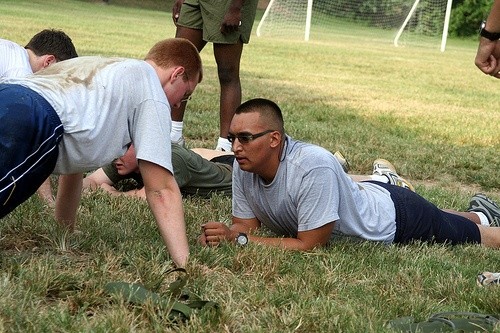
left=235, top=232, right=248, bottom=248
left=479, top=20, right=500, bottom=41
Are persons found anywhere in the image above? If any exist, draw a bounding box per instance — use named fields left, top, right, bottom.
left=169, top=0, right=259, bottom=152
left=199, top=98, right=500, bottom=250
left=0, top=29, right=80, bottom=208
left=82, top=142, right=416, bottom=200
left=473, top=0, right=500, bottom=79
left=0, top=37, right=205, bottom=277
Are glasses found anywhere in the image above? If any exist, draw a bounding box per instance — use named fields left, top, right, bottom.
left=227, top=129, right=276, bottom=144
left=175, top=66, right=192, bottom=103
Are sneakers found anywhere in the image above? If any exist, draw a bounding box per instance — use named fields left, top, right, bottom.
left=468, top=192, right=500, bottom=227
left=335, top=150, right=350, bottom=173
left=372, top=158, right=416, bottom=193
left=477, top=271, right=500, bottom=287
